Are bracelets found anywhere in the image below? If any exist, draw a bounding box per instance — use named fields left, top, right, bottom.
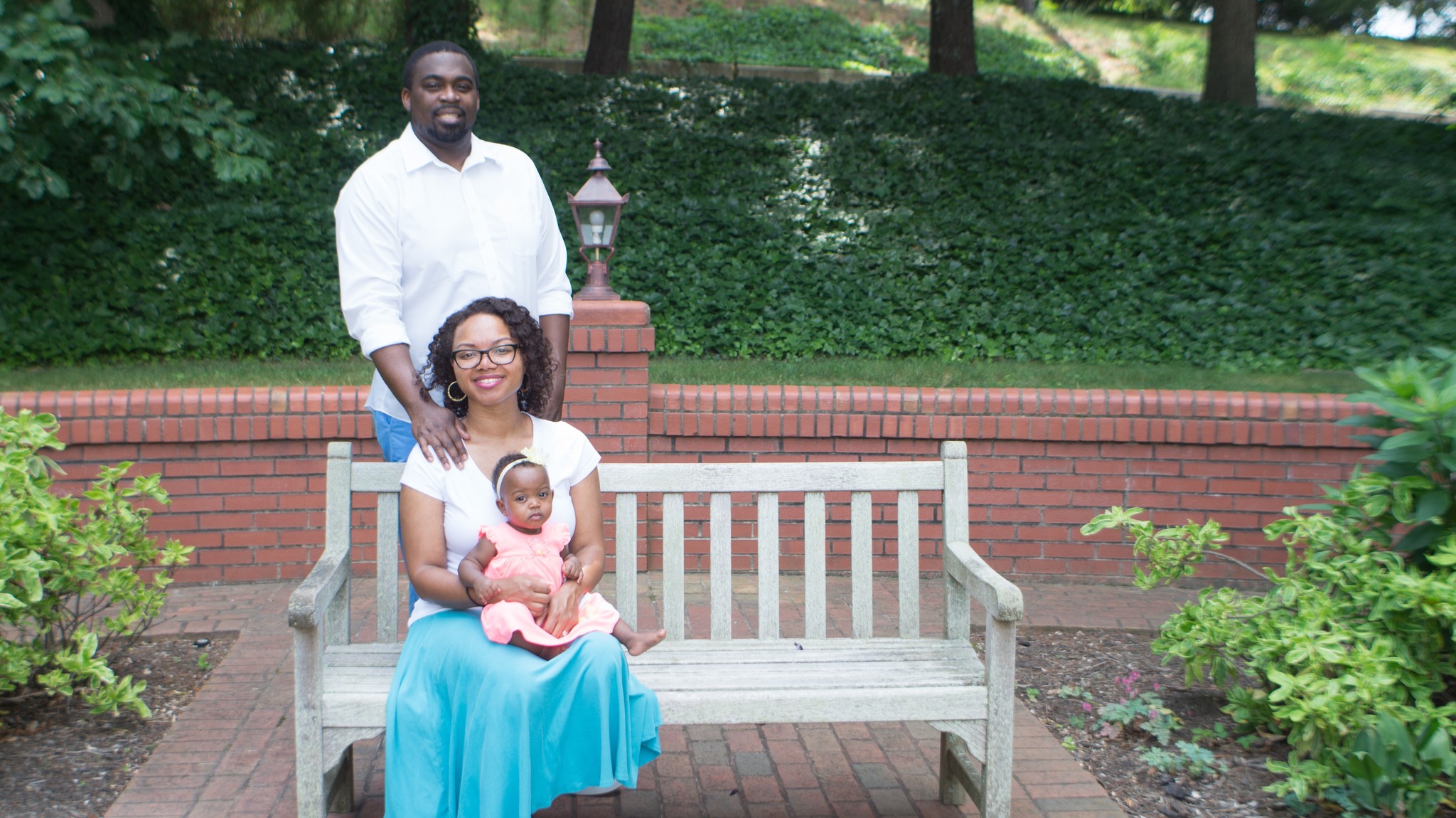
left=466, top=586, right=486, bottom=608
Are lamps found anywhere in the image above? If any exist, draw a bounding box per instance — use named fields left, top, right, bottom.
left=565, top=133, right=631, bottom=298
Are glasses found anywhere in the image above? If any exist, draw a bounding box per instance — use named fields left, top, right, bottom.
left=451, top=344, right=523, bottom=369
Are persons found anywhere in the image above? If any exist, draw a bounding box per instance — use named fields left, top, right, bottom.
left=334, top=39, right=572, bottom=621
left=457, top=448, right=668, bottom=662
left=387, top=295, right=661, bottom=818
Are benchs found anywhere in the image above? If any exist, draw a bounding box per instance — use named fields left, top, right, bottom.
left=286, top=438, right=1022, bottom=818
